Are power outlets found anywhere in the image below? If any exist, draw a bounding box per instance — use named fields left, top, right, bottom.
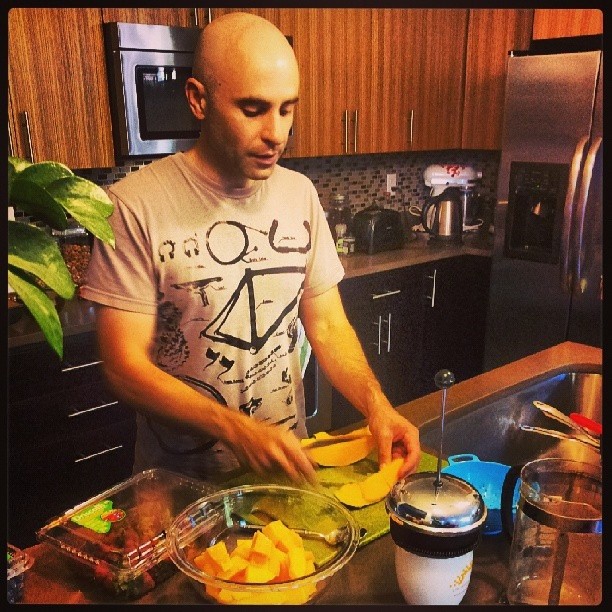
left=386, top=174, right=397, bottom=199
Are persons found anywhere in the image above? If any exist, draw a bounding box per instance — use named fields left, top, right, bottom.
left=77, top=11, right=422, bottom=491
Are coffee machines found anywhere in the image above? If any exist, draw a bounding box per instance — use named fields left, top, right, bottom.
left=426, top=182, right=485, bottom=231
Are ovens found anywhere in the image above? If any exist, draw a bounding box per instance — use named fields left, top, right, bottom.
left=305, top=354, right=333, bottom=434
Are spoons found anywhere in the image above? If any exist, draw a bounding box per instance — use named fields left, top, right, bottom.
left=245, top=523, right=366, bottom=548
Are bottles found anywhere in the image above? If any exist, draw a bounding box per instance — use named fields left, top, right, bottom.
left=328, top=193, right=354, bottom=246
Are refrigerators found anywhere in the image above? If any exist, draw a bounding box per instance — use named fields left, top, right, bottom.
left=483, top=49, right=604, bottom=368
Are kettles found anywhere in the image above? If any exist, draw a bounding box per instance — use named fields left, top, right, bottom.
left=422, top=186, right=463, bottom=244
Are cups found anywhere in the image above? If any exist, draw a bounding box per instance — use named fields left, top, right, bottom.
left=384, top=473, right=488, bottom=605
left=499, top=460, right=602, bottom=609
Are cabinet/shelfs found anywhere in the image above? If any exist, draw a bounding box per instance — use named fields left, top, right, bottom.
left=334, top=261, right=421, bottom=430
left=462, top=10, right=532, bottom=152
left=7, top=6, right=117, bottom=170
left=289, top=5, right=373, bottom=166
left=102, top=7, right=290, bottom=39
left=421, top=255, right=491, bottom=377
left=373, top=7, right=469, bottom=156
left=1, top=329, right=132, bottom=542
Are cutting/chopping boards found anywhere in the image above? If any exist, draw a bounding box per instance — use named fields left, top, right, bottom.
left=214, top=447, right=449, bottom=551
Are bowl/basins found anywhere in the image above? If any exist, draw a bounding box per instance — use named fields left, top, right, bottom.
left=166, top=483, right=360, bottom=605
left=438, top=453, right=539, bottom=533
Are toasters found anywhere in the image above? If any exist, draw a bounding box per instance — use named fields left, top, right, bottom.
left=354, top=207, right=404, bottom=255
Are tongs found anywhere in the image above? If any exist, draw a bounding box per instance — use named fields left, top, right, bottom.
left=519, top=401, right=600, bottom=451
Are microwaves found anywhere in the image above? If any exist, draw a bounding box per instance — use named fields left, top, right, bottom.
left=105, top=21, right=294, bottom=156
